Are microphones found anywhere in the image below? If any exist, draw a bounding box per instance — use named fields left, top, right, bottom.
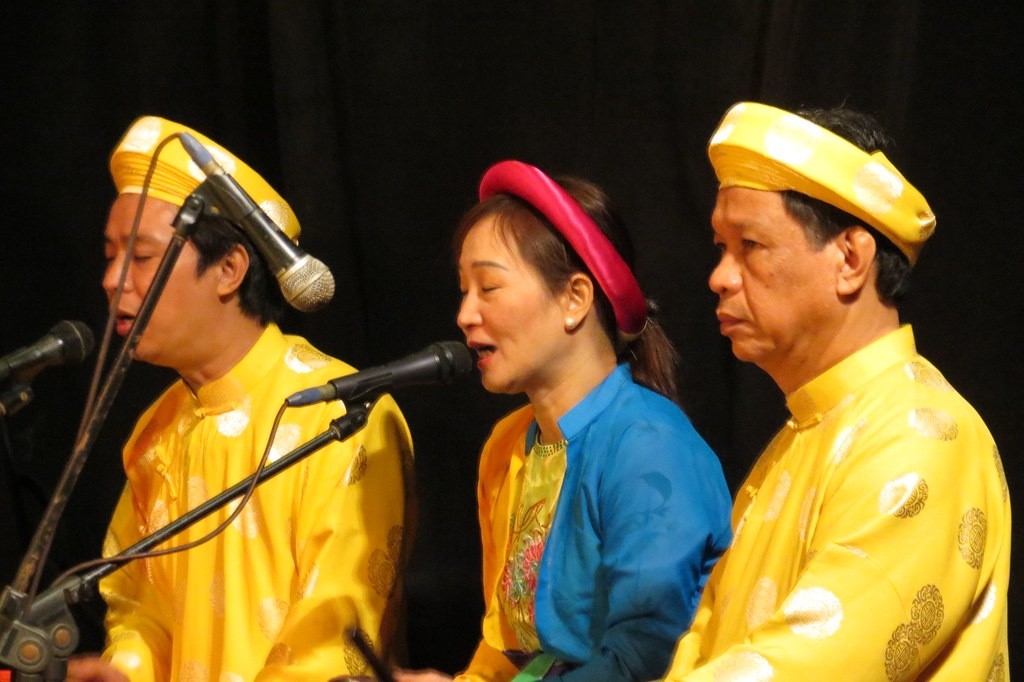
left=181, top=133, right=335, bottom=313
left=287, top=342, right=473, bottom=407
left=0, top=320, right=94, bottom=393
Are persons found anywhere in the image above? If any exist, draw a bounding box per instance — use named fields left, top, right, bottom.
left=64, top=117, right=412, bottom=682
left=394, top=162, right=733, bottom=682
left=653, top=102, right=1011, bottom=682
